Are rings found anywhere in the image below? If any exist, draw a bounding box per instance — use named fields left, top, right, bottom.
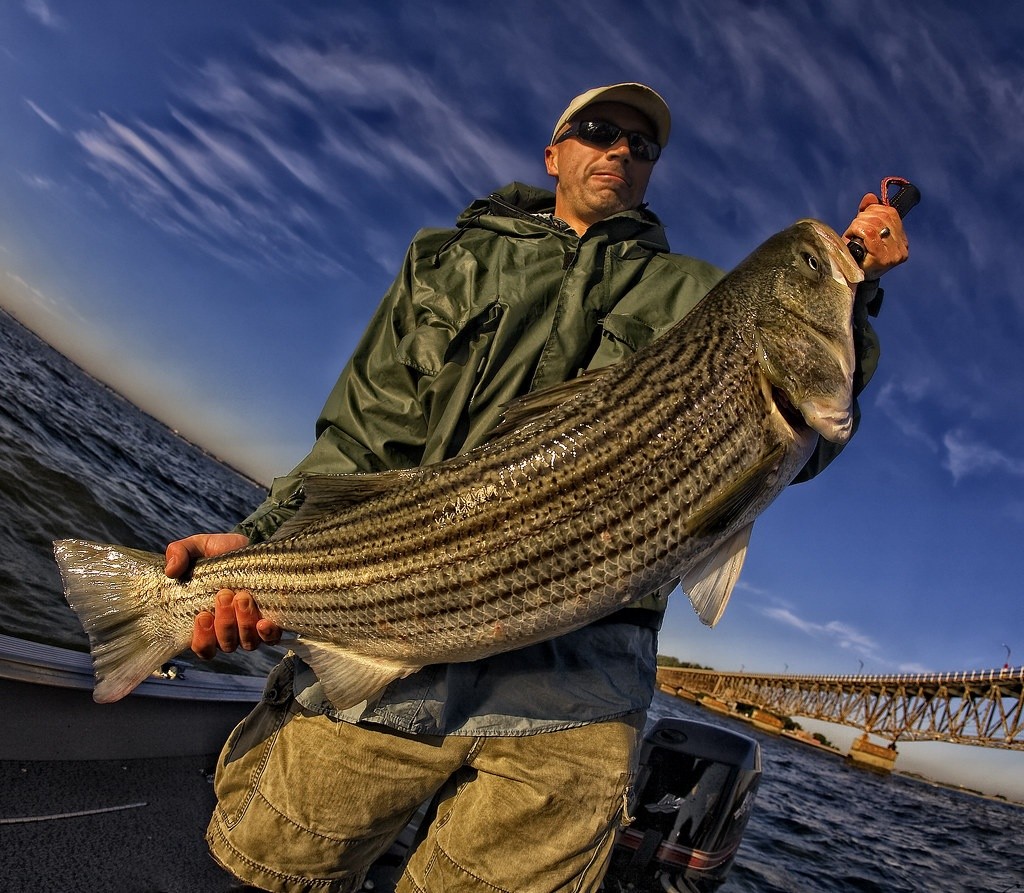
left=879, top=227, right=889, bottom=237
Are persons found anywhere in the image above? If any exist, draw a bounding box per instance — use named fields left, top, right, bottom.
left=165, top=83, right=909, bottom=893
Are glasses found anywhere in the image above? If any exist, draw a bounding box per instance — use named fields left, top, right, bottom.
left=554, top=116, right=662, bottom=163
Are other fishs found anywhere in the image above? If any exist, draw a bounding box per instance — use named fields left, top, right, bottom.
left=52, top=214, right=867, bottom=710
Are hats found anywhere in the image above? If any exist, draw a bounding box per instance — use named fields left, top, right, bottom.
left=550, top=81, right=672, bottom=151
